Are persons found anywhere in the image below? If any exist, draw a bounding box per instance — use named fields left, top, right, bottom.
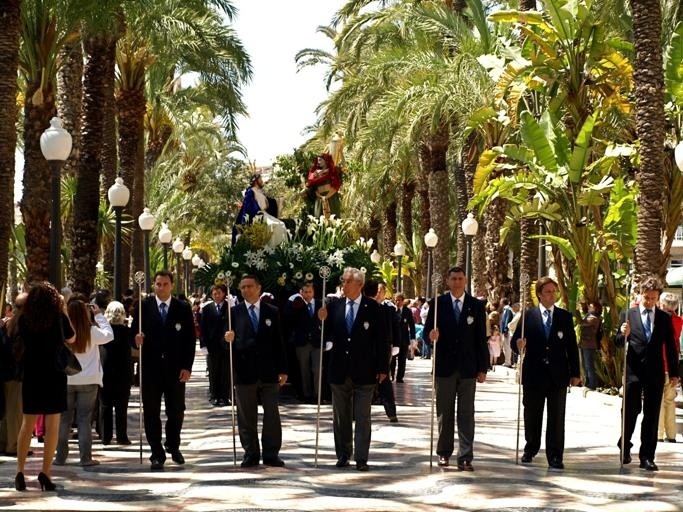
left=232, top=170, right=294, bottom=230
left=574, top=295, right=604, bottom=391
left=510, top=277, right=579, bottom=469
left=374, top=283, right=432, bottom=384
left=221, top=275, right=288, bottom=469
left=0, top=265, right=136, bottom=493
left=658, top=292, right=683, bottom=443
left=320, top=267, right=392, bottom=470
left=423, top=267, right=492, bottom=472
left=198, top=282, right=320, bottom=407
left=614, top=276, right=681, bottom=471
left=300, top=130, right=350, bottom=216
left=478, top=297, right=523, bottom=367
left=131, top=271, right=196, bottom=467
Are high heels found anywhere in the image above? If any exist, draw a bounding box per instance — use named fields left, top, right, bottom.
left=37, top=472, right=57, bottom=493
left=13, top=472, right=27, bottom=493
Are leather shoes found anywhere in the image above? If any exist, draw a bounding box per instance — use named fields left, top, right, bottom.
left=618, top=446, right=632, bottom=464
left=520, top=447, right=539, bottom=463
left=208, top=391, right=231, bottom=406
left=335, top=456, right=370, bottom=472
left=457, top=459, right=475, bottom=472
left=241, top=453, right=286, bottom=469
left=421, top=355, right=432, bottom=360
left=396, top=379, right=404, bottom=383
left=548, top=456, right=565, bottom=472
left=389, top=416, right=398, bottom=422
left=437, top=453, right=451, bottom=466
left=164, top=440, right=186, bottom=464
left=100, top=438, right=131, bottom=445
left=150, top=458, right=164, bottom=471
left=640, top=457, right=658, bottom=471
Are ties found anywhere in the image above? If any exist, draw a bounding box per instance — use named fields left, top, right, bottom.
left=308, top=302, right=314, bottom=316
left=215, top=305, right=222, bottom=313
left=452, top=298, right=463, bottom=327
left=246, top=306, right=263, bottom=331
left=643, top=311, right=654, bottom=342
left=158, top=302, right=170, bottom=321
left=345, top=300, right=355, bottom=334
left=543, top=311, right=553, bottom=335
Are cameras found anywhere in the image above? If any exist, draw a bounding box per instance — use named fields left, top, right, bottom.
left=123, top=318, right=128, bottom=324
left=87, top=305, right=95, bottom=309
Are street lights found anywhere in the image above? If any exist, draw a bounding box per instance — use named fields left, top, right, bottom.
left=370, top=248, right=380, bottom=265
left=137, top=206, right=155, bottom=296
left=460, top=212, right=479, bottom=298
left=108, top=177, right=130, bottom=303
left=530, top=190, right=552, bottom=280
left=158, top=221, right=206, bottom=298
left=393, top=240, right=405, bottom=292
left=39, top=117, right=74, bottom=294
left=424, top=227, right=438, bottom=300
left=673, top=141, right=682, bottom=172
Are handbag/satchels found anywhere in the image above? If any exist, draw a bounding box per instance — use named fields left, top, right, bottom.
left=52, top=345, right=81, bottom=376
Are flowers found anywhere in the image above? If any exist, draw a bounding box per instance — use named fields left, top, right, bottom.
left=192, top=209, right=417, bottom=300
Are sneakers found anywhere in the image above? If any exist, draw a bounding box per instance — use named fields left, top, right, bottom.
left=51, top=460, right=100, bottom=466
left=658, top=436, right=677, bottom=443
